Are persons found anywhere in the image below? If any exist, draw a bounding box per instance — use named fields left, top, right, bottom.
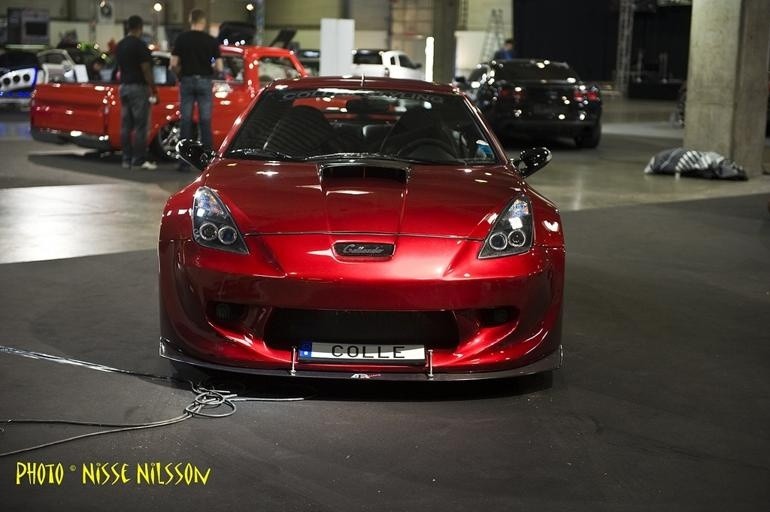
left=493, top=40, right=516, bottom=60
left=114, top=15, right=159, bottom=170
left=169, top=8, right=224, bottom=172
left=57, top=31, right=75, bottom=49
left=87, top=57, right=105, bottom=81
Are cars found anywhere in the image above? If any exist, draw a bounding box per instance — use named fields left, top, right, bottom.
left=354, top=44, right=429, bottom=80
left=0, top=38, right=319, bottom=163
left=158, top=75, right=566, bottom=382
left=452, top=58, right=605, bottom=153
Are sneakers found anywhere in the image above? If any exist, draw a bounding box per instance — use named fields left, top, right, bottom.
left=122, top=162, right=131, bottom=169
left=132, top=160, right=158, bottom=171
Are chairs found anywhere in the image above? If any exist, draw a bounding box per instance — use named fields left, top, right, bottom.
left=261, top=104, right=461, bottom=161
